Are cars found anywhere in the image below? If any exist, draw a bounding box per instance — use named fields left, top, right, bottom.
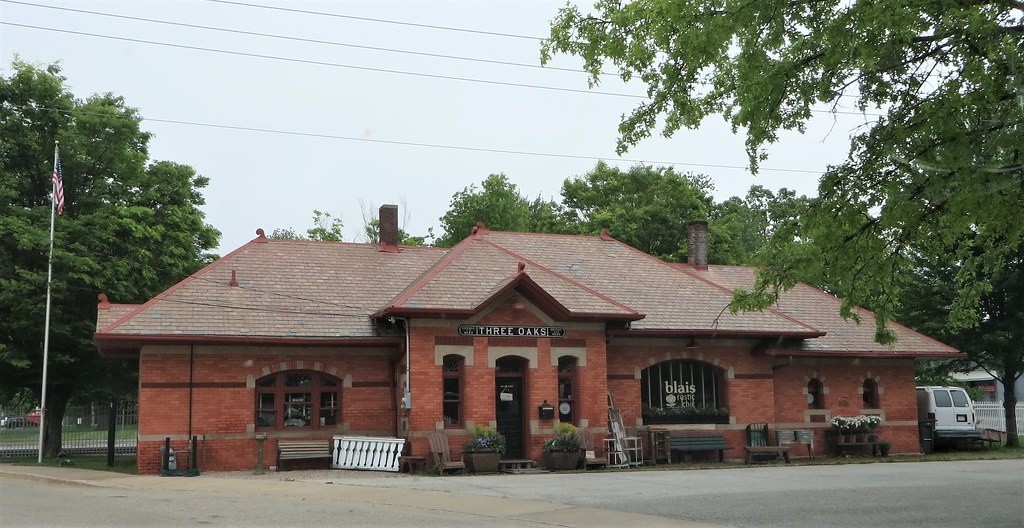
left=3, top=415, right=38, bottom=429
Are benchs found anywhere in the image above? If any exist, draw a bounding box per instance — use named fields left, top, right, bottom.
left=670, top=434, right=735, bottom=463
left=276, top=439, right=334, bottom=472
left=499, top=459, right=534, bottom=470
left=745, top=446, right=792, bottom=465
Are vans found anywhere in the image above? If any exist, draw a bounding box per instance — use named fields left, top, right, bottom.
left=916, top=386, right=982, bottom=450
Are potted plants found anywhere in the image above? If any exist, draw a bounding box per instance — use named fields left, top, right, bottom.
left=642, top=403, right=729, bottom=425
left=878, top=440, right=891, bottom=457
left=543, top=422, right=583, bottom=470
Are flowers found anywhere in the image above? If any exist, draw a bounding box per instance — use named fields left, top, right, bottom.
left=464, top=422, right=506, bottom=456
left=845, top=417, right=862, bottom=435
left=867, top=414, right=884, bottom=435
left=830, top=415, right=851, bottom=435
left=854, top=414, right=871, bottom=435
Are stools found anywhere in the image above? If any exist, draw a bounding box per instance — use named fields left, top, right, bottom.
left=398, top=456, right=427, bottom=475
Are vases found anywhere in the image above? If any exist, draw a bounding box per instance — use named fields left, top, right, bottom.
left=459, top=450, right=502, bottom=474
left=834, top=435, right=845, bottom=443
left=845, top=435, right=856, bottom=443
left=869, top=435, right=880, bottom=442
left=857, top=435, right=868, bottom=443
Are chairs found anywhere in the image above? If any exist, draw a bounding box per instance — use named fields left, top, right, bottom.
left=427, top=431, right=465, bottom=477
left=576, top=427, right=607, bottom=471
left=746, top=421, right=780, bottom=465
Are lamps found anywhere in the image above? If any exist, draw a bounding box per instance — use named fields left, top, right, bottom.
left=686, top=335, right=702, bottom=349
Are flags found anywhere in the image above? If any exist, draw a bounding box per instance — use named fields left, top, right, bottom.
left=52, top=148, right=64, bottom=216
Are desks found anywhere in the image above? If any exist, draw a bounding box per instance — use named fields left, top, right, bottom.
left=830, top=442, right=881, bottom=458
left=777, top=440, right=816, bottom=461
